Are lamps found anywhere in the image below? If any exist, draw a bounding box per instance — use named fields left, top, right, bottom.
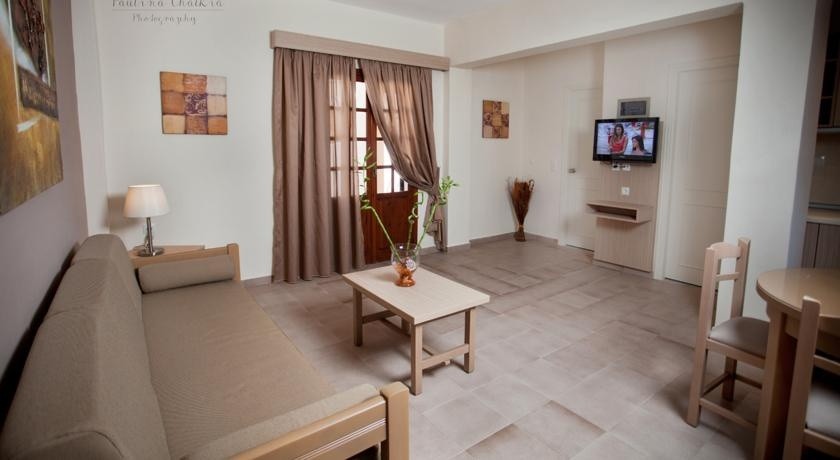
left=123, top=185, right=169, bottom=257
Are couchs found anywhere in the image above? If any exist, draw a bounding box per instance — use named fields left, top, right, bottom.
left=5, top=232, right=408, bottom=459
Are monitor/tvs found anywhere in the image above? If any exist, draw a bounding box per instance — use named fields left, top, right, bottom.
left=592, top=117, right=659, bottom=165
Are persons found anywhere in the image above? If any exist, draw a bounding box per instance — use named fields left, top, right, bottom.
left=631, top=134, right=652, bottom=155
left=608, top=123, right=628, bottom=156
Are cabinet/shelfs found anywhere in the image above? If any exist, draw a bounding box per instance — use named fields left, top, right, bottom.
left=586, top=201, right=653, bottom=223
left=804, top=219, right=840, bottom=270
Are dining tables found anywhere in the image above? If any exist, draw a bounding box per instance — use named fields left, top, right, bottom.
left=756, top=267, right=839, bottom=459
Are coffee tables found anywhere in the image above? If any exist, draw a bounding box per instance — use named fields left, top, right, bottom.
left=341, top=263, right=491, bottom=396
left=125, top=245, right=205, bottom=256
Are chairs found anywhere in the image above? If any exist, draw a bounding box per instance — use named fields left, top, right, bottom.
left=779, top=296, right=839, bottom=460
left=685, top=236, right=790, bottom=435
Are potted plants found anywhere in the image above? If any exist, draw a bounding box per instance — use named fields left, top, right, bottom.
left=359, top=148, right=459, bottom=287
